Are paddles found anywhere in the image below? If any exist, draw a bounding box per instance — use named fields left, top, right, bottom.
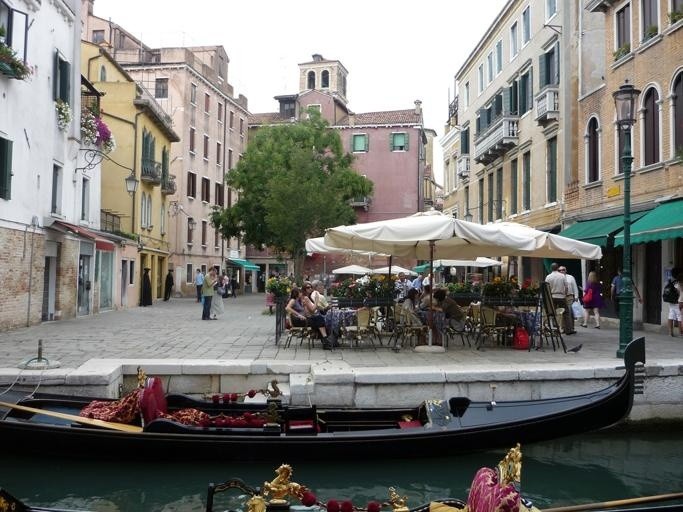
left=541, top=492, right=681, bottom=510
left=1, top=402, right=143, bottom=435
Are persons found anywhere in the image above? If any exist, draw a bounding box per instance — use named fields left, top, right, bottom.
left=576, top=269, right=601, bottom=329
left=283, top=272, right=539, bottom=351
left=198, top=267, right=217, bottom=321
left=558, top=265, right=579, bottom=334
left=209, top=267, right=225, bottom=320
left=609, top=266, right=643, bottom=320
left=192, top=268, right=236, bottom=303
left=666, top=267, right=682, bottom=338
left=544, top=262, right=577, bottom=336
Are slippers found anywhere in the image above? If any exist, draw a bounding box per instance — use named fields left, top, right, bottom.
left=581, top=323, right=587, bottom=327
left=595, top=325, right=600, bottom=328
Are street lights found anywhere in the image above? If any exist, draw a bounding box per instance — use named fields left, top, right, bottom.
left=612, top=79, right=641, bottom=358
left=125, top=169, right=137, bottom=234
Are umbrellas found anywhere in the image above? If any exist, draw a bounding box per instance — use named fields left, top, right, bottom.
left=483, top=221, right=603, bottom=262
left=331, top=257, right=504, bottom=277
left=302, top=236, right=391, bottom=266
left=320, top=207, right=534, bottom=342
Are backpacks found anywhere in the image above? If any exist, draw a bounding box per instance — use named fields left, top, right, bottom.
left=663, top=279, right=679, bottom=303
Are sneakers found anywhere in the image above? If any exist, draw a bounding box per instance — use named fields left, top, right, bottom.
left=322, top=336, right=332, bottom=345
left=324, top=343, right=332, bottom=350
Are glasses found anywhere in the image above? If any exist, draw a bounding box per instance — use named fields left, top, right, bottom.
left=306, top=285, right=313, bottom=289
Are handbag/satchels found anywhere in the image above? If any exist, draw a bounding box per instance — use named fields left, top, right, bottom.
left=581, top=289, right=593, bottom=303
left=218, top=287, right=225, bottom=295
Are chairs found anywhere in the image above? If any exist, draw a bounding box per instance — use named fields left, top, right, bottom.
left=284, top=304, right=565, bottom=354
left=137, top=376, right=166, bottom=427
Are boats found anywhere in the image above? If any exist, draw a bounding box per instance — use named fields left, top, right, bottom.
left=1, top=337, right=648, bottom=467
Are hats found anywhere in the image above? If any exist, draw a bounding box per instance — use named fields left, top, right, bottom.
left=434, top=289, right=444, bottom=298
left=408, top=288, right=416, bottom=296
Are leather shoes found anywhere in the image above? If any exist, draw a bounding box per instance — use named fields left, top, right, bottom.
left=567, top=330, right=576, bottom=335
left=202, top=316, right=209, bottom=320
